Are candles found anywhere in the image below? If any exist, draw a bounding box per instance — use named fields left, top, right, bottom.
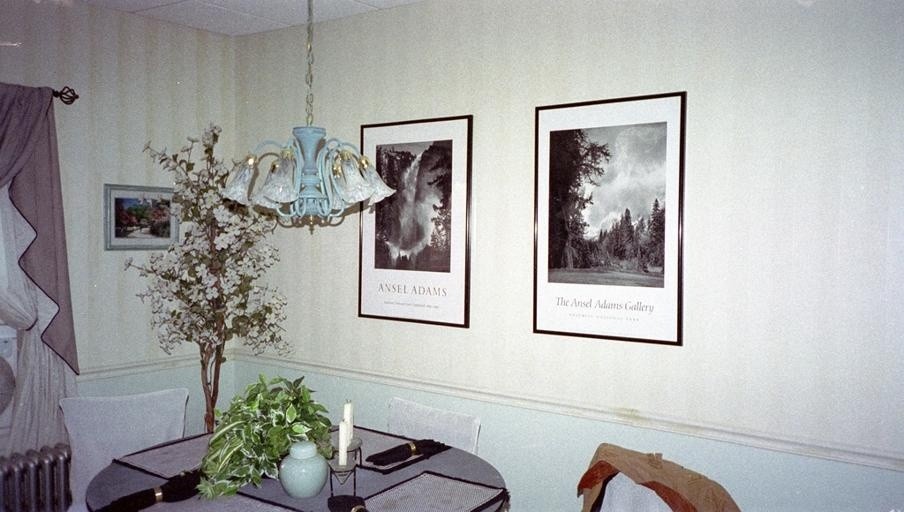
left=343, top=401, right=354, bottom=442
left=336, top=420, right=348, bottom=465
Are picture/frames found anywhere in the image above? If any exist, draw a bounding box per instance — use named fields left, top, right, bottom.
left=356, top=113, right=474, bottom=329
left=532, top=90, right=689, bottom=348
left=101, top=181, right=180, bottom=252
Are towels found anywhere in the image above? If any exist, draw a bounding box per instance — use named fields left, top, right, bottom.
left=326, top=494, right=368, bottom=512
left=94, top=471, right=203, bottom=511
left=367, top=438, right=448, bottom=468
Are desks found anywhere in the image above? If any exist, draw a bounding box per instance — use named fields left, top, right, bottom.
left=86, top=421, right=510, bottom=512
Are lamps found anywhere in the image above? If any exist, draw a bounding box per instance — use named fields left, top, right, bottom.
left=221, top=0, right=396, bottom=231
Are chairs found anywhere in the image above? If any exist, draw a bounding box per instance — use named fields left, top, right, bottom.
left=577, top=441, right=742, bottom=511
left=57, top=387, right=190, bottom=512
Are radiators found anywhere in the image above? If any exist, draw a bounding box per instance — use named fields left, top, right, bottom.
left=0, top=444, right=73, bottom=512
